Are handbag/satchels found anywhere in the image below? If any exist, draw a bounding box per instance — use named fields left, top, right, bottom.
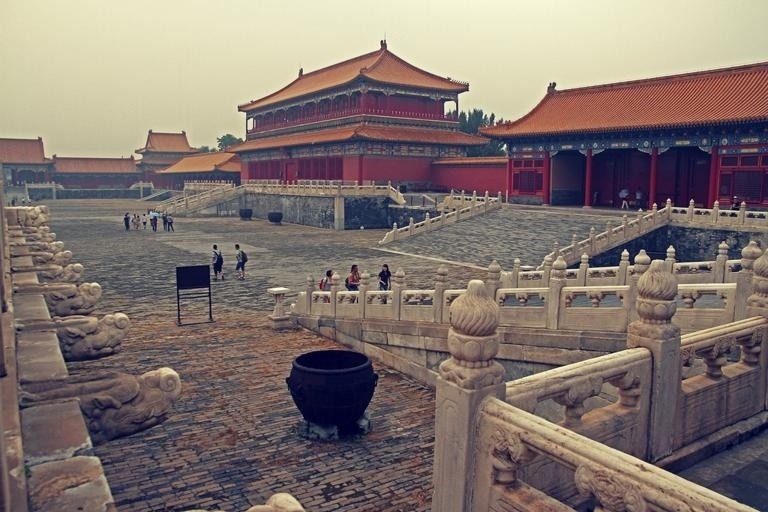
left=344, top=278, right=348, bottom=287
left=378, top=282, right=381, bottom=288
left=320, top=279, right=327, bottom=289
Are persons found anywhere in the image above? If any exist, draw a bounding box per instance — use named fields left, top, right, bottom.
left=322, top=269, right=338, bottom=303
left=121, top=209, right=175, bottom=233
left=634, top=187, right=643, bottom=209
left=211, top=244, right=225, bottom=281
left=728, top=196, right=741, bottom=217
left=347, top=264, right=361, bottom=303
left=233, top=244, right=245, bottom=280
left=376, top=264, right=392, bottom=304
left=11, top=194, right=33, bottom=206
left=619, top=186, right=629, bottom=210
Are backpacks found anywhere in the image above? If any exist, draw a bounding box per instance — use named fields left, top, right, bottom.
left=213, top=250, right=223, bottom=265
left=238, top=250, right=247, bottom=262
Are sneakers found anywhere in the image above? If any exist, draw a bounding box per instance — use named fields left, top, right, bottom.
left=213, top=276, right=224, bottom=280
